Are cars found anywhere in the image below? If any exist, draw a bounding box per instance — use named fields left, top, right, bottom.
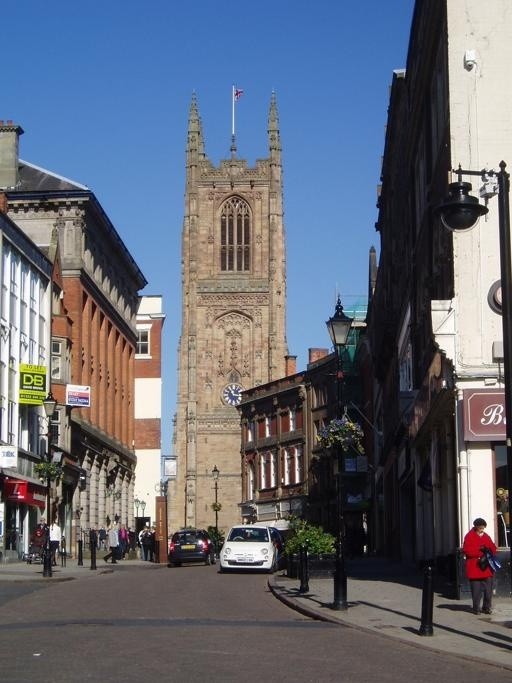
left=269, top=526, right=285, bottom=568
left=219, top=525, right=279, bottom=574
left=169, top=529, right=217, bottom=566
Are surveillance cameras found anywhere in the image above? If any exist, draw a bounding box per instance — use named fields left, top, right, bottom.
left=80, top=476, right=85, bottom=480
left=465, top=50, right=474, bottom=68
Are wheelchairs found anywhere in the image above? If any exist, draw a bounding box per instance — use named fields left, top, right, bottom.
left=26, top=542, right=48, bottom=564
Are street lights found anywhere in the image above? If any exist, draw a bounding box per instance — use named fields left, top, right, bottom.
left=141, top=500, right=146, bottom=518
left=435, top=157, right=511, bottom=558
left=212, top=464, right=219, bottom=559
left=134, top=498, right=140, bottom=517
left=323, top=290, right=353, bottom=609
left=41, top=392, right=58, bottom=577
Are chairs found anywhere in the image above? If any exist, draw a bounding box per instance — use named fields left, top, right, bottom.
left=497, top=512, right=510, bottom=547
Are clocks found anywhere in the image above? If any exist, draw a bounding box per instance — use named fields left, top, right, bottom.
left=221, top=383, right=245, bottom=407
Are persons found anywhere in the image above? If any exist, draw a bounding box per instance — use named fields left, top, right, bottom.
left=463, top=518, right=497, bottom=615
left=27, top=517, right=156, bottom=568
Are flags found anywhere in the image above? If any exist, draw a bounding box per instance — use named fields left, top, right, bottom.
left=235, top=89, right=243, bottom=101
